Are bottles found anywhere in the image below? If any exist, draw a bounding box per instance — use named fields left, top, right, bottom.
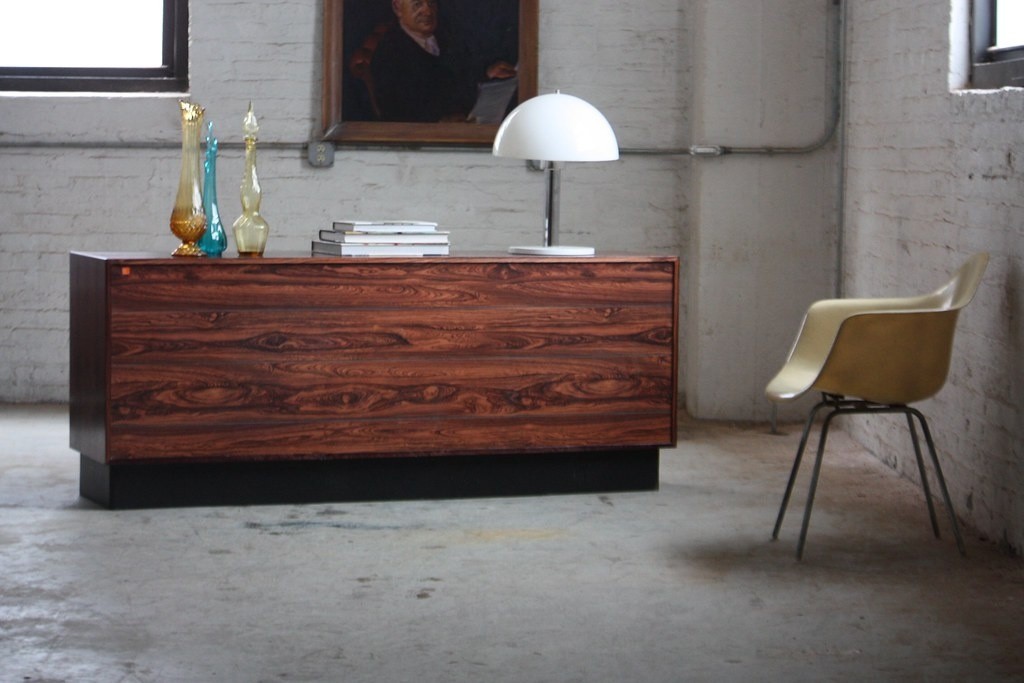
left=233, top=103, right=269, bottom=257
left=171, top=99, right=228, bottom=259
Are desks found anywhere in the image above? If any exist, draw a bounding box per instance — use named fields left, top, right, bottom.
left=69, top=248, right=680, bottom=508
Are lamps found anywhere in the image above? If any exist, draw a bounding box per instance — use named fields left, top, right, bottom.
left=492, top=89, right=620, bottom=256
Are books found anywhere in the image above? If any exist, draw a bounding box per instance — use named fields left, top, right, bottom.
left=311, top=221, right=450, bottom=256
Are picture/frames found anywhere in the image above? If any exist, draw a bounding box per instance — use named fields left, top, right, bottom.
left=322, top=0, right=540, bottom=146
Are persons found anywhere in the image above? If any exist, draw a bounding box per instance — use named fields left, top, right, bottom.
left=370, top=0, right=517, bottom=123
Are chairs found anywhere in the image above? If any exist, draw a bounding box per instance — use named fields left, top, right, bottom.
left=761, top=250, right=991, bottom=562
